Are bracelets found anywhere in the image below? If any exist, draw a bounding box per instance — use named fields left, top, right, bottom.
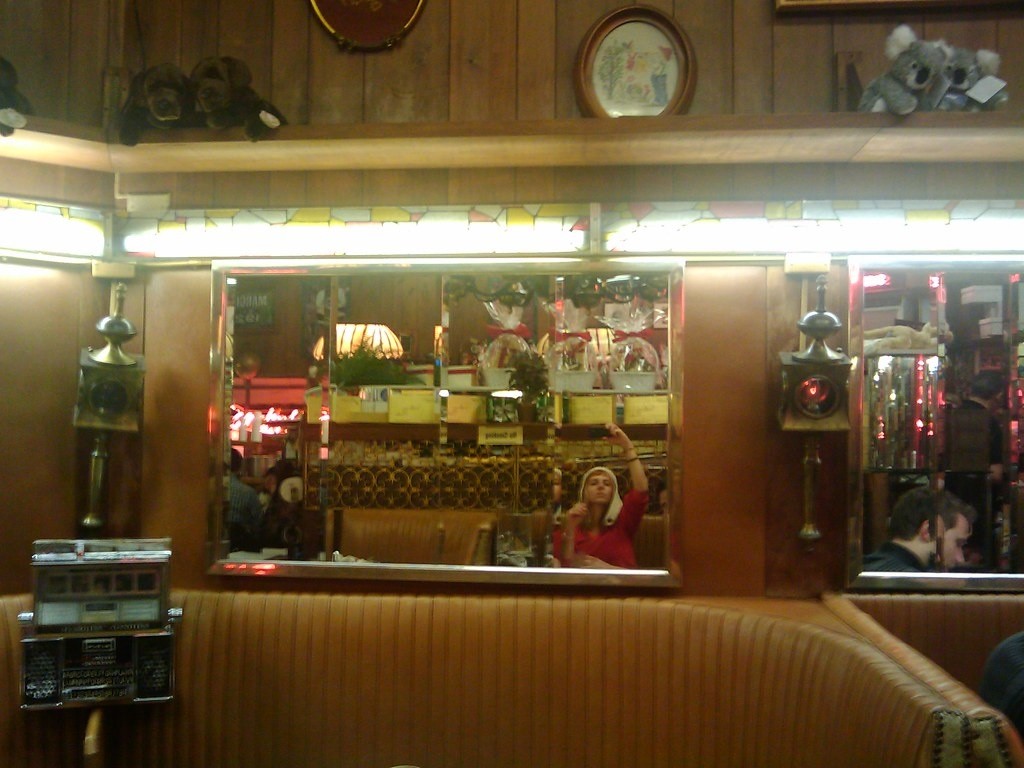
left=623, top=447, right=634, bottom=453
left=627, top=457, right=639, bottom=463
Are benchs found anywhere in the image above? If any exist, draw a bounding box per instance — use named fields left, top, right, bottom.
left=0, top=591, right=971, bottom=768
left=818, top=591, right=1024, bottom=768
left=493, top=513, right=667, bottom=570
left=323, top=508, right=496, bottom=566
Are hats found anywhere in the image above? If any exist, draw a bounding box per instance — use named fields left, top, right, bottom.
left=578, top=466, right=623, bottom=526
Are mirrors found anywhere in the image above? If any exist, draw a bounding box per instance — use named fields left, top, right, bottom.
left=202, top=257, right=686, bottom=589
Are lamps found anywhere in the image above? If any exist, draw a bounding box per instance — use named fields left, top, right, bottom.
left=563, top=272, right=666, bottom=310
left=442, top=272, right=534, bottom=307
left=312, top=323, right=403, bottom=362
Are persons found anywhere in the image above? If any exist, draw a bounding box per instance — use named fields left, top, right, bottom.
left=973, top=629, right=1024, bottom=744
left=552, top=420, right=650, bottom=569
left=945, top=367, right=1006, bottom=564
left=848, top=487, right=977, bottom=586
left=227, top=450, right=327, bottom=560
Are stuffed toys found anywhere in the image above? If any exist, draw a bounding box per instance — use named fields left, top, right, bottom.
left=0, top=55, right=32, bottom=136
left=857, top=24, right=1009, bottom=114
left=862, top=318, right=952, bottom=352
left=118, top=58, right=286, bottom=146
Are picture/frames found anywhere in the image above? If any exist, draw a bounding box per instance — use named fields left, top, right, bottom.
left=845, top=253, right=1024, bottom=594
left=571, top=4, right=697, bottom=118
left=652, top=302, right=668, bottom=330
left=309, top=0, right=425, bottom=54
